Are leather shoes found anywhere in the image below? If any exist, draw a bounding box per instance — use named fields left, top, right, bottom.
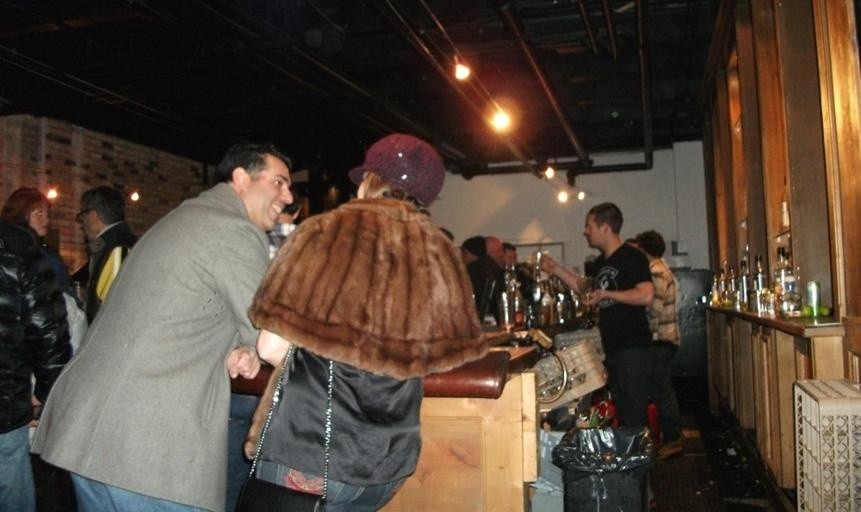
left=657, top=446, right=683, bottom=460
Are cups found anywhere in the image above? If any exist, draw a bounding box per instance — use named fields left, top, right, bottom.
left=806, top=280, right=821, bottom=318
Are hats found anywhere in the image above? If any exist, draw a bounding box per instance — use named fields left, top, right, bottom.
left=463, top=236, right=486, bottom=258
left=349, top=134, right=445, bottom=206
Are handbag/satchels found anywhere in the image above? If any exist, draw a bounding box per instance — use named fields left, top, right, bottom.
left=236, top=476, right=327, bottom=511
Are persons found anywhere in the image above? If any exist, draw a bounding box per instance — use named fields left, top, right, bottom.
left=3, top=186, right=81, bottom=307
left=228, top=131, right=491, bottom=511
left=1, top=214, right=74, bottom=511
left=459, top=234, right=533, bottom=323
left=537, top=201, right=681, bottom=433
left=29, top=139, right=296, bottom=512
left=68, top=184, right=139, bottom=324
left=266, top=196, right=302, bottom=260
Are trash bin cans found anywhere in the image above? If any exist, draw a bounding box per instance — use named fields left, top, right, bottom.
left=552, top=426, right=654, bottom=512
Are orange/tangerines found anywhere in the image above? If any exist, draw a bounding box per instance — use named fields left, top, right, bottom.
left=818, top=304, right=831, bottom=317
left=799, top=304, right=814, bottom=317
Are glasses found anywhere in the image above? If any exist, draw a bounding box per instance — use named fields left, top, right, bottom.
left=75, top=209, right=93, bottom=224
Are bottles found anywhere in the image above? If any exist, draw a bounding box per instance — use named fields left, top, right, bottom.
left=754, top=254, right=766, bottom=290
left=494, top=248, right=580, bottom=334
left=774, top=247, right=797, bottom=296
left=709, top=264, right=736, bottom=307
left=740, top=262, right=750, bottom=311
